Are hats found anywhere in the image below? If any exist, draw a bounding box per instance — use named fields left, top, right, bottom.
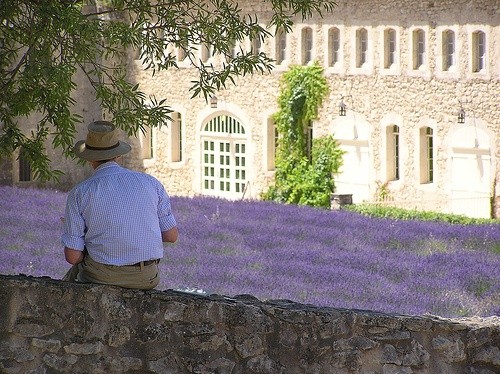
left=74, top=120, right=132, bottom=160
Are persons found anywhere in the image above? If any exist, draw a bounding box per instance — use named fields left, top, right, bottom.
left=59, top=120, right=179, bottom=290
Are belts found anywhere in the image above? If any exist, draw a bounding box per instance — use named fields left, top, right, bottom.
left=126, top=261, right=155, bottom=266
